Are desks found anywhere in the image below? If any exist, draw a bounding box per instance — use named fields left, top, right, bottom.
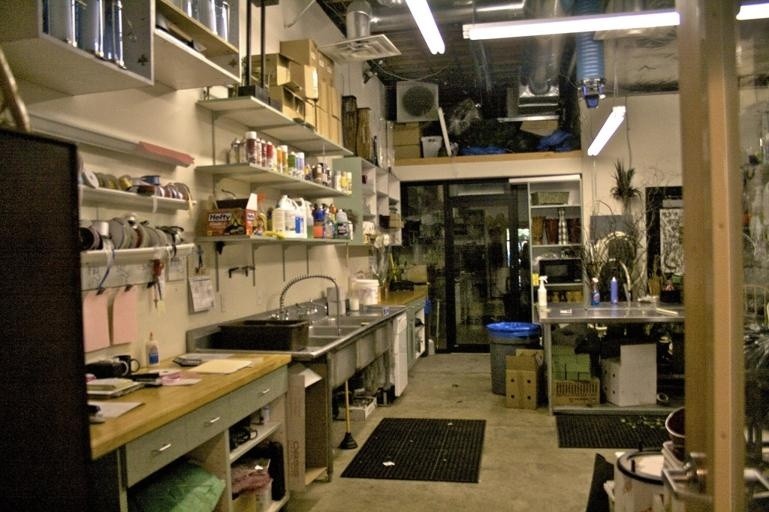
left=535, top=301, right=684, bottom=415
left=85, top=350, right=291, bottom=512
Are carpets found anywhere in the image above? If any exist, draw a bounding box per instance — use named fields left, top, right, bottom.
left=340, top=417, right=485, bottom=484
left=554, top=412, right=683, bottom=450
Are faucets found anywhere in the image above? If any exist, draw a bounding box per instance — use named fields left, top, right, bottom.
left=307, top=297, right=330, bottom=316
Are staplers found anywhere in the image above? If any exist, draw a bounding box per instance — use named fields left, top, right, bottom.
left=88, top=405, right=106, bottom=424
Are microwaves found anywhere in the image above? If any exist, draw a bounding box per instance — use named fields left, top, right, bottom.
left=539, top=258, right=582, bottom=284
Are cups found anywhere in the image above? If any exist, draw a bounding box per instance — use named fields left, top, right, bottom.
left=98, top=355, right=140, bottom=377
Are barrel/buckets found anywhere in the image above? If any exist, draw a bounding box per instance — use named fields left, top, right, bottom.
left=665, top=408, right=685, bottom=459
left=354, top=279, right=378, bottom=305
left=278, top=194, right=307, bottom=239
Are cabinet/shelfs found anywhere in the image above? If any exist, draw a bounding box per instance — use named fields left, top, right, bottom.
left=375, top=284, right=428, bottom=370
left=526, top=176, right=586, bottom=323
left=0, top=0, right=401, bottom=289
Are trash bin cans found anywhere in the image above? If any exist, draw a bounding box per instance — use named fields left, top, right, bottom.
left=486, top=322, right=542, bottom=395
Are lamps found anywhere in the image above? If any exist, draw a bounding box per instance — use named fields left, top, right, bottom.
left=461, top=0, right=768, bottom=40
left=586, top=43, right=627, bottom=156
left=403, top=0, right=445, bottom=55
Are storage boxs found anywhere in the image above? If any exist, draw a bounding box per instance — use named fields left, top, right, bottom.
left=504, top=349, right=545, bottom=410
left=599, top=343, right=657, bottom=407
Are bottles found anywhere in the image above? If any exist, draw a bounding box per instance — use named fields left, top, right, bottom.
left=146, top=332, right=159, bottom=367
left=228, top=132, right=353, bottom=195
left=337, top=210, right=349, bottom=239
left=546, top=290, right=581, bottom=303
left=610, top=277, right=619, bottom=304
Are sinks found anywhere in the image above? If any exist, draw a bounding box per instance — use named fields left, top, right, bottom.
left=307, top=337, right=338, bottom=346
left=309, top=325, right=359, bottom=337
left=320, top=314, right=373, bottom=324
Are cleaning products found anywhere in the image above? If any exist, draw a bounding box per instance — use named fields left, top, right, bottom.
left=538, top=276, right=549, bottom=307
left=592, top=277, right=602, bottom=303
